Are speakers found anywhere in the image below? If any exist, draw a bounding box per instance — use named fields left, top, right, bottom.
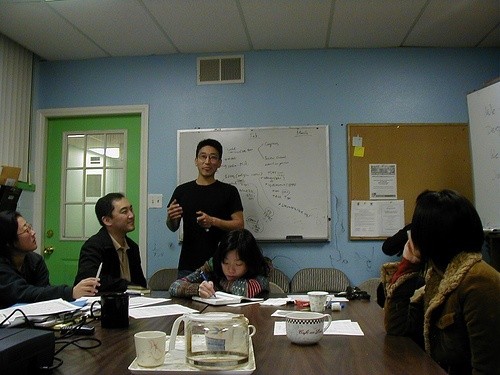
left=100, top=292, right=129, bottom=330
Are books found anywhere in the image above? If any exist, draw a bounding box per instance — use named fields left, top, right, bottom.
left=258, top=292, right=330, bottom=306
left=192, top=291, right=262, bottom=306
left=126, top=285, right=151, bottom=293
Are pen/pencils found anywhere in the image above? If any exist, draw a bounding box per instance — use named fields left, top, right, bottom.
left=91, top=261, right=103, bottom=293
left=201, top=271, right=216, bottom=298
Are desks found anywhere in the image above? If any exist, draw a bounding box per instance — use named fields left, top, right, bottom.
left=48, top=290, right=447, bottom=375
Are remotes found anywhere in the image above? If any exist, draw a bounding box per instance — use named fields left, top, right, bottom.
left=59, top=326, right=95, bottom=336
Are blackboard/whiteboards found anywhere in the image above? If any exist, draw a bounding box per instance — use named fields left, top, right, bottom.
left=465, top=77, right=499, bottom=231
left=180, top=125, right=329, bottom=240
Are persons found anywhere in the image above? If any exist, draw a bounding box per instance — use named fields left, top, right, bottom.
left=166, top=139, right=245, bottom=279
left=0, top=210, right=101, bottom=307
left=381, top=189, right=500, bottom=375
left=73, top=192, right=147, bottom=293
left=168, top=229, right=275, bottom=298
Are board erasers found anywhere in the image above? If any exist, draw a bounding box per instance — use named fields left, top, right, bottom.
left=287, top=235, right=304, bottom=240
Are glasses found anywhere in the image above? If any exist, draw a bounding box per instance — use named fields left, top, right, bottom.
left=198, top=153, right=219, bottom=160
left=17, top=223, right=32, bottom=235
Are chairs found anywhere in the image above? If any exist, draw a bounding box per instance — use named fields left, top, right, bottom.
left=148, top=269, right=179, bottom=291
left=269, top=268, right=382, bottom=302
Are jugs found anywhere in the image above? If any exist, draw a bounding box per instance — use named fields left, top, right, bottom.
left=168, top=313, right=250, bottom=371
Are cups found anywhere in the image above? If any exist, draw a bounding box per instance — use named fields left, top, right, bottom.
left=286, top=312, right=332, bottom=345
left=204, top=312, right=256, bottom=354
left=307, top=291, right=332, bottom=313
left=134, top=330, right=171, bottom=368
left=90, top=293, right=129, bottom=329
